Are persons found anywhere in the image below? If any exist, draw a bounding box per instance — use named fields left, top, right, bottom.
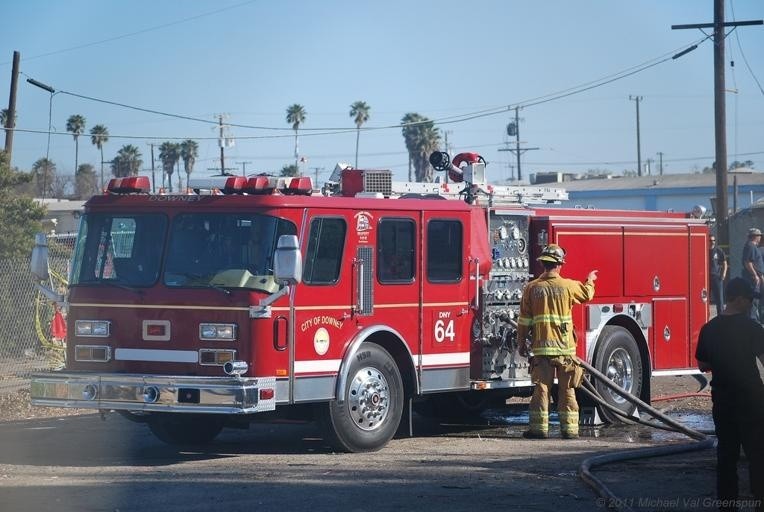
left=741, top=227, right=763, bottom=327
left=515, top=243, right=599, bottom=438
left=709, top=234, right=728, bottom=315
left=697, top=274, right=764, bottom=511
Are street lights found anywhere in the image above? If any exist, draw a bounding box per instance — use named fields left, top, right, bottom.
left=628, top=95, right=645, bottom=177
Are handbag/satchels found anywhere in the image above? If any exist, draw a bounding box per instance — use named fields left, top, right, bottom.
left=568, top=366, right=586, bottom=389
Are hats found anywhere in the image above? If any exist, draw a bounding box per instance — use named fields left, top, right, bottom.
left=726, top=277, right=762, bottom=299
left=747, top=228, right=764, bottom=237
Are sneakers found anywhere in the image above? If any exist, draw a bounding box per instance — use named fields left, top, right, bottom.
left=561, top=429, right=580, bottom=439
left=522, top=428, right=550, bottom=439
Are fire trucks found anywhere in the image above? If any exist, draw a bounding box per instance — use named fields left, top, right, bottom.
left=28, top=152, right=711, bottom=456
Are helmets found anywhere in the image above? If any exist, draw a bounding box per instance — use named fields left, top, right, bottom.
left=535, top=243, right=566, bottom=265
